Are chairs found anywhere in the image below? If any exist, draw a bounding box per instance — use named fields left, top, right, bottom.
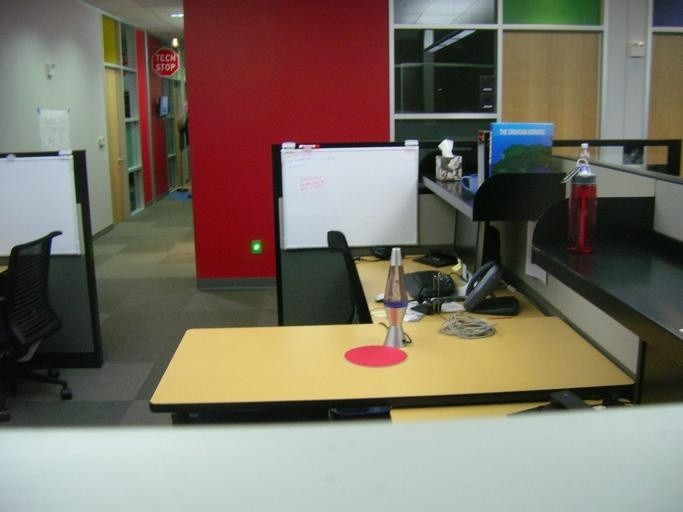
left=328, top=231, right=389, bottom=419
left=1, top=230, right=72, bottom=422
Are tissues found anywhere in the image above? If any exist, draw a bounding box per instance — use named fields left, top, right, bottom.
left=433, top=140, right=464, bottom=182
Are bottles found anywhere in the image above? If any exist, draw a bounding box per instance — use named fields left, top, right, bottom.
left=567, top=159, right=597, bottom=253
left=574, top=142, right=593, bottom=174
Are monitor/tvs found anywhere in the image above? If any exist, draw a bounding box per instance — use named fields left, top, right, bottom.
left=451, top=209, right=501, bottom=284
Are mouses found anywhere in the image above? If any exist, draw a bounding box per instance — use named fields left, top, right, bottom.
left=375, top=293, right=384, bottom=302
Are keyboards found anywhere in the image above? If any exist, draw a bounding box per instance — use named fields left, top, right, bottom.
left=404, top=269, right=454, bottom=298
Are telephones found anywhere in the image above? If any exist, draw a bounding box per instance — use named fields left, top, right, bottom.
left=461, top=261, right=520, bottom=314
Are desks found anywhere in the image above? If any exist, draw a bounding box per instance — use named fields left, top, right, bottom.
left=149, top=317, right=640, bottom=424
left=353, top=249, right=634, bottom=419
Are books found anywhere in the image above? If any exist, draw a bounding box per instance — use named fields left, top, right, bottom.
left=476, top=122, right=555, bottom=188
